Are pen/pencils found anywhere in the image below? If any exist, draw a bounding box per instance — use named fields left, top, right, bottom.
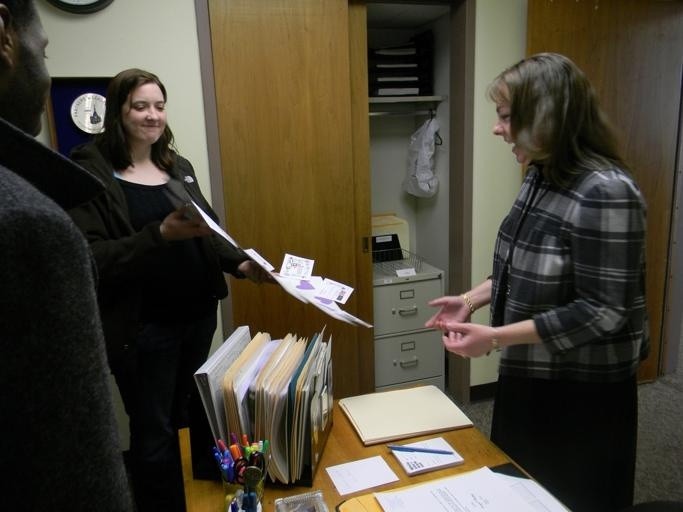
left=384, top=444, right=453, bottom=457
left=211, top=431, right=270, bottom=485
left=229, top=491, right=258, bottom=512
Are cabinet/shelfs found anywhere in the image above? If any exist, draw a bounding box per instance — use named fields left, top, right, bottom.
left=373, top=261, right=446, bottom=393
left=197, top=1, right=473, bottom=408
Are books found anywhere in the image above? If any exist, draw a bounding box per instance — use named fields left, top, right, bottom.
left=193, top=324, right=334, bottom=487
left=373, top=45, right=422, bottom=95
left=392, top=438, right=466, bottom=475
left=338, top=383, right=474, bottom=447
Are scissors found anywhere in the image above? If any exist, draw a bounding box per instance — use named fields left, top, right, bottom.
left=233, top=451, right=266, bottom=484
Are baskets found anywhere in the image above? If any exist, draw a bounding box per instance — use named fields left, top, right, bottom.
left=372, top=248, right=424, bottom=276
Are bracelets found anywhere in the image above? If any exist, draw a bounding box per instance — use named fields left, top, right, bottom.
left=460, top=293, right=475, bottom=313
left=492, top=331, right=503, bottom=353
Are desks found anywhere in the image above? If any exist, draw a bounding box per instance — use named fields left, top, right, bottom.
left=176, top=382, right=573, bottom=511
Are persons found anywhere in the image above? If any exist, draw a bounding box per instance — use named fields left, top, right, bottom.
left=67, top=68, right=278, bottom=451
left=423, top=50, right=648, bottom=512
left=0, top=1, right=138, bottom=511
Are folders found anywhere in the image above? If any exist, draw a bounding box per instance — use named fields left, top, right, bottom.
left=193, top=323, right=253, bottom=446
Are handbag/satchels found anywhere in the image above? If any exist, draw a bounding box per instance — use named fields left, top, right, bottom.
left=405, top=119, right=440, bottom=198
